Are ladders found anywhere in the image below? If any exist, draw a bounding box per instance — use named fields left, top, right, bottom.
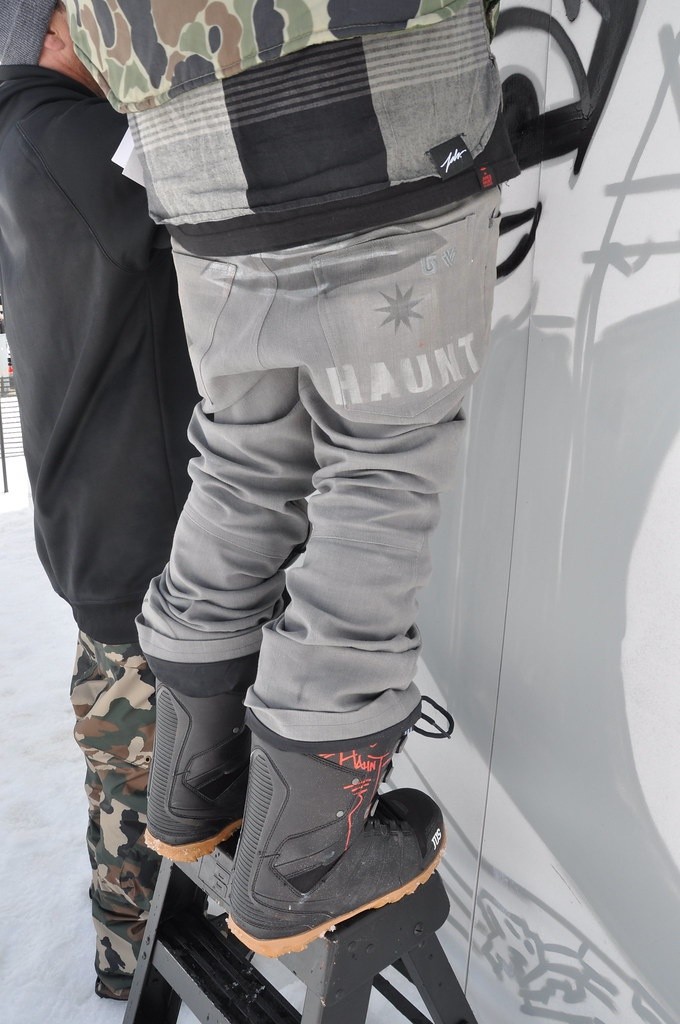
left=124, top=826, right=478, bottom=1023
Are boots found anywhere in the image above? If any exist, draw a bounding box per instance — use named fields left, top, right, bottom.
left=145, top=677, right=246, bottom=863
left=226, top=696, right=454, bottom=958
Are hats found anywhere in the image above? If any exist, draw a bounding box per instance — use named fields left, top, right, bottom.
left=0, top=0, right=58, bottom=66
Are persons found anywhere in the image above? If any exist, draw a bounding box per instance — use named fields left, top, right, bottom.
left=59, top=0, right=521, bottom=958
left=0, top=0, right=209, bottom=1001
